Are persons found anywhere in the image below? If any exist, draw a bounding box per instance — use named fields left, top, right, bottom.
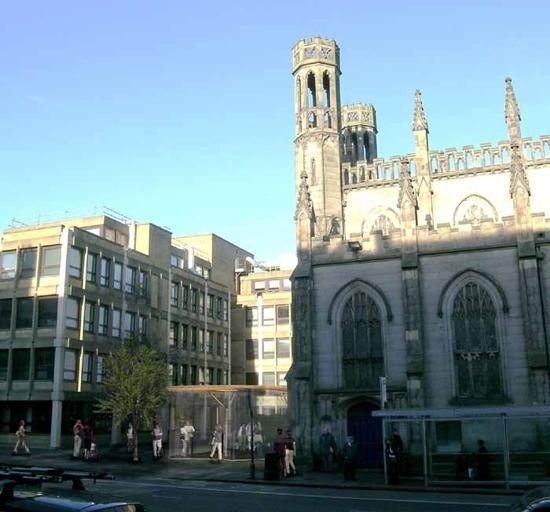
left=389, top=428, right=403, bottom=452
left=245, top=422, right=256, bottom=450
left=340, top=433, right=361, bottom=482
left=283, top=430, right=296, bottom=475
left=319, top=427, right=337, bottom=473
left=13, top=418, right=32, bottom=455
left=180, top=418, right=196, bottom=456
left=238, top=422, right=247, bottom=451
left=72, top=419, right=84, bottom=457
left=271, top=428, right=286, bottom=478
left=128, top=423, right=134, bottom=452
left=254, top=422, right=263, bottom=449
left=473, top=439, right=492, bottom=482
left=149, top=422, right=164, bottom=457
left=83, top=421, right=95, bottom=459
left=384, top=437, right=403, bottom=485
left=209, top=425, right=223, bottom=461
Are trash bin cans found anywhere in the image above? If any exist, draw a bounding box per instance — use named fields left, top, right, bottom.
left=265, top=453, right=280, bottom=480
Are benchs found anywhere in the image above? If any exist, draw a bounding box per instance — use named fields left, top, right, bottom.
left=429, top=451, right=549, bottom=486
